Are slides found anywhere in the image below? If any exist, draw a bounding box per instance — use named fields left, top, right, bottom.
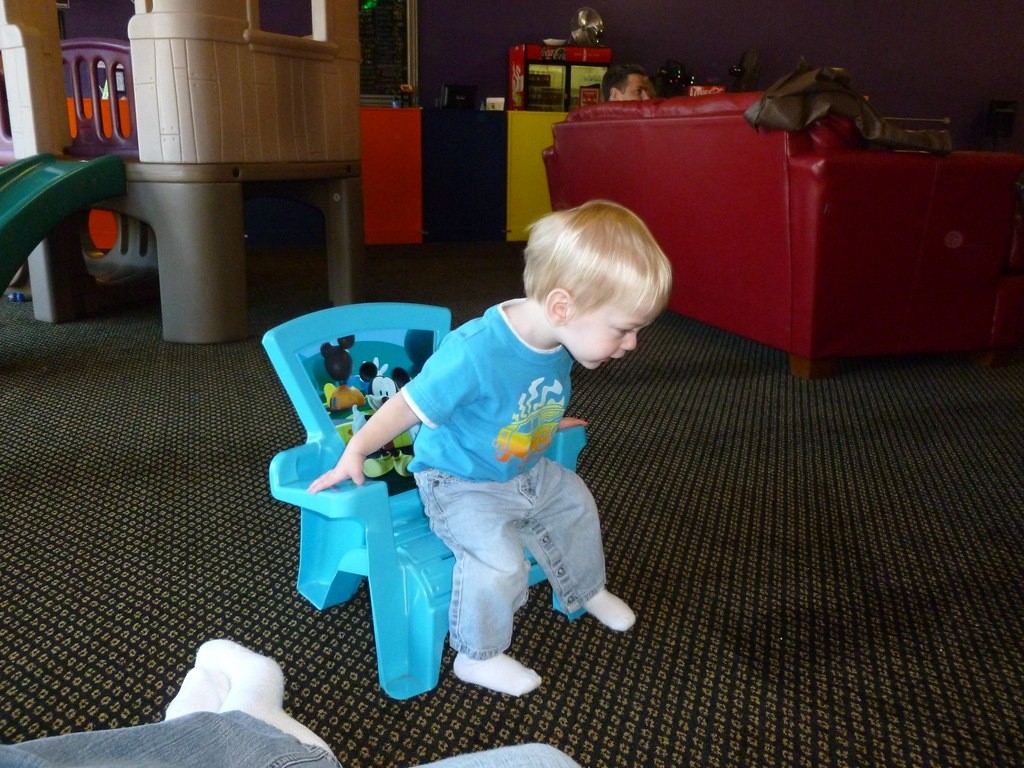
left=0, top=152, right=130, bottom=298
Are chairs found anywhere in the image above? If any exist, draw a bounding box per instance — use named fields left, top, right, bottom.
left=260, top=302, right=586, bottom=699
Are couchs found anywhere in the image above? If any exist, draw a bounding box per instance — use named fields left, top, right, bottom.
left=541, top=92, right=1024, bottom=384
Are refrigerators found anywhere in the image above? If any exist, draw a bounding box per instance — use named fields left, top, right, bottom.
left=509, top=44, right=612, bottom=112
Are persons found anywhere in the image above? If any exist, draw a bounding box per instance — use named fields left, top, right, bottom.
left=601, top=63, right=656, bottom=102
left=308, top=201, right=672, bottom=696
left=0, top=639, right=582, bottom=767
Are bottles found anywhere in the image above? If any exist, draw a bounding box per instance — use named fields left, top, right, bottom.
left=526, top=69, right=561, bottom=107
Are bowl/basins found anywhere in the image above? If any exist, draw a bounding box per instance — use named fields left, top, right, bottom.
left=544, top=39, right=567, bottom=46
